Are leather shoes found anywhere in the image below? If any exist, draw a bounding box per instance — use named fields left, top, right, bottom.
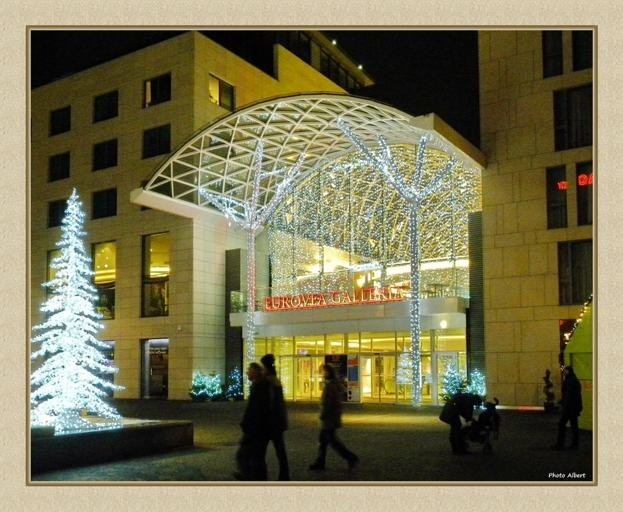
left=549, top=442, right=581, bottom=451
left=310, top=458, right=326, bottom=471
left=343, top=457, right=360, bottom=472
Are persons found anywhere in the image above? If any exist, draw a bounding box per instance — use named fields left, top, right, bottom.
left=309, top=362, right=358, bottom=472
left=559, top=366, right=583, bottom=450
left=234, top=353, right=290, bottom=481
left=439, top=395, right=495, bottom=458
